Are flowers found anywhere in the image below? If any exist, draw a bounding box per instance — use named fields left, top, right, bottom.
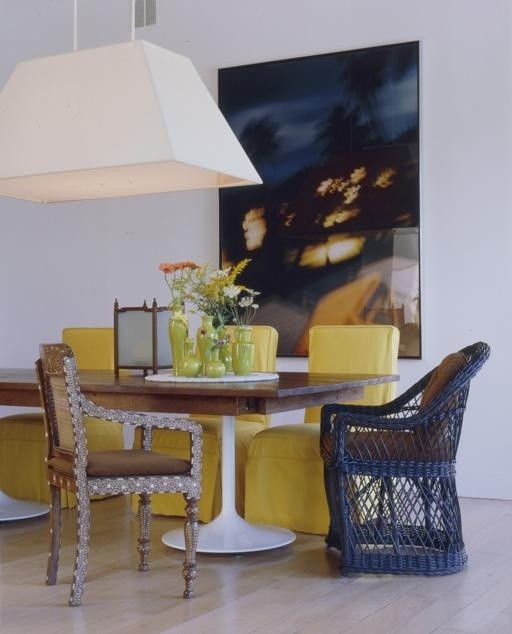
left=162, top=257, right=262, bottom=326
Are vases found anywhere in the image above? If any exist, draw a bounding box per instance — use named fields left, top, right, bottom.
left=168, top=306, right=188, bottom=375
left=196, top=315, right=217, bottom=376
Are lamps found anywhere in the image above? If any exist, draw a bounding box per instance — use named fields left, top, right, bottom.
left=0, top=0, right=264, bottom=205
